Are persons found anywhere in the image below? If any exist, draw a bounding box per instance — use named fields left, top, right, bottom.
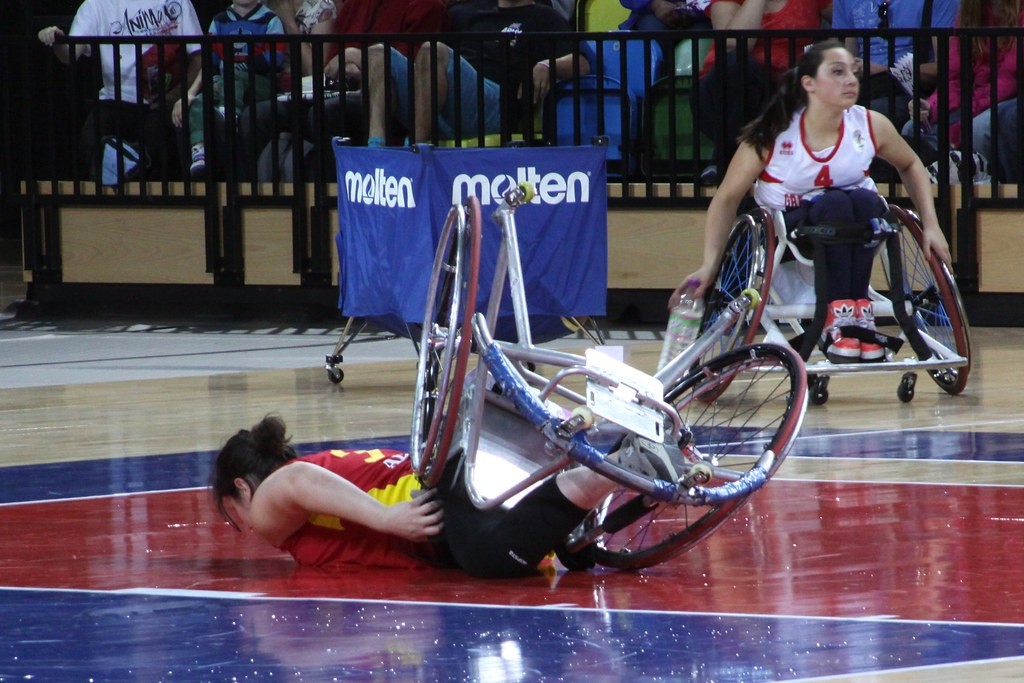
left=173, top=0, right=338, bottom=180
left=38, top=1, right=205, bottom=186
left=240, top=1, right=1023, bottom=182
left=213, top=417, right=684, bottom=580
left=668, top=42, right=954, bottom=363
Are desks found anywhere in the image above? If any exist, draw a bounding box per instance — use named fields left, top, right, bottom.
left=326, top=137, right=607, bottom=397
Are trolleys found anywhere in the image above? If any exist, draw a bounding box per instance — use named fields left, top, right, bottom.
left=325, top=133, right=605, bottom=385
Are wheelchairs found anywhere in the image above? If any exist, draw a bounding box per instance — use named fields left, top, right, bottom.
left=689, top=204, right=971, bottom=405
left=408, top=179, right=807, bottom=571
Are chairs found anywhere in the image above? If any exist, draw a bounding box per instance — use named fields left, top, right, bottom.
left=552, top=27, right=715, bottom=161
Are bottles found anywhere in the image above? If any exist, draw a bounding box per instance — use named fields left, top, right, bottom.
left=656, top=276, right=706, bottom=387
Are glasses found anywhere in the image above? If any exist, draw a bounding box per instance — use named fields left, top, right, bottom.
left=877, top=1, right=890, bottom=30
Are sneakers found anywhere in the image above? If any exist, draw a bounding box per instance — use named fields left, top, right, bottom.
left=855, top=298, right=885, bottom=359
left=618, top=430, right=687, bottom=484
left=821, top=299, right=861, bottom=358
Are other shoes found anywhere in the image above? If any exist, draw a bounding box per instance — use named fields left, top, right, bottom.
left=700, top=166, right=718, bottom=187
left=189, top=146, right=206, bottom=175
